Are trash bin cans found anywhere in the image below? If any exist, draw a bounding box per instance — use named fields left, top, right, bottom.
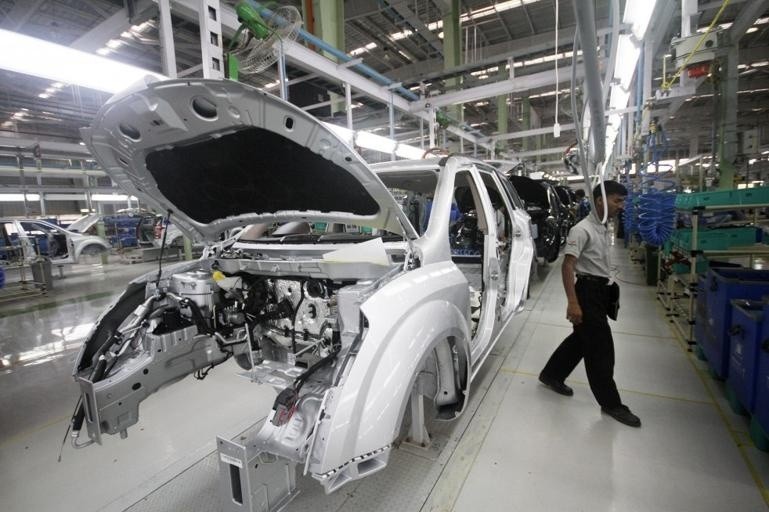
left=31, top=255, right=52, bottom=291
left=694, top=260, right=769, bottom=436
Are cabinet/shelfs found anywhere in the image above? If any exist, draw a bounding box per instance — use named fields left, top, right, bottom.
left=656, top=203, right=769, bottom=353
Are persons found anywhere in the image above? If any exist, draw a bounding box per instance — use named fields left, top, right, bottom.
left=575, top=188, right=590, bottom=224
left=536, top=180, right=642, bottom=428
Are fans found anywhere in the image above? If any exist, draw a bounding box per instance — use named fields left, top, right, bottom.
left=225, top=1, right=302, bottom=75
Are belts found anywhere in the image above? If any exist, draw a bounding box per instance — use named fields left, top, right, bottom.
left=574, top=273, right=610, bottom=283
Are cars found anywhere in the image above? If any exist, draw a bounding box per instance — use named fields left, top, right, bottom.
left=0, top=215, right=112, bottom=270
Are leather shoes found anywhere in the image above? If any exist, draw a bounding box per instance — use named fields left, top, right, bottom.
left=600, top=402, right=643, bottom=429
left=538, top=370, right=574, bottom=397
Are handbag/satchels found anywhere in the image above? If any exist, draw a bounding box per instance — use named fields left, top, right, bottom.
left=599, top=281, right=621, bottom=322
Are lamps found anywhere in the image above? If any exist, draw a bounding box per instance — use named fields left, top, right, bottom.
left=605, top=0, right=657, bottom=158
left=0, top=29, right=433, bottom=159
